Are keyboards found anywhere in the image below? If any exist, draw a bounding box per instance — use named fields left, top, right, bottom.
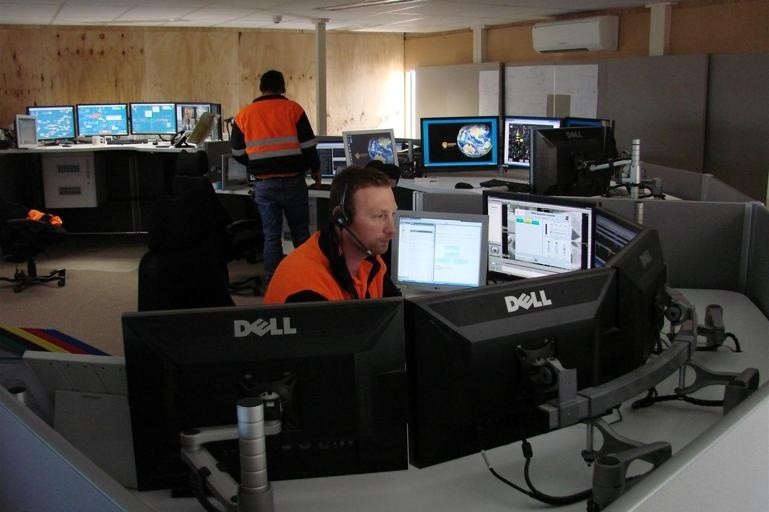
left=480, top=179, right=530, bottom=193
left=107, top=139, right=148, bottom=144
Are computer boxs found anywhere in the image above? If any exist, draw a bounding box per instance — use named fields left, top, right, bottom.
left=178, top=103, right=222, bottom=140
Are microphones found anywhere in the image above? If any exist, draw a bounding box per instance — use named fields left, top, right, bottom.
left=335, top=218, right=373, bottom=257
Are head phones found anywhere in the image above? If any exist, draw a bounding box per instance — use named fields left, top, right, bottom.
left=333, top=168, right=380, bottom=227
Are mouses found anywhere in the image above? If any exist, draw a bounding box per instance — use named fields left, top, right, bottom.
left=454, top=182, right=473, bottom=189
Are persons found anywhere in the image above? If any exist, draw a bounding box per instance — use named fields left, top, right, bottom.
left=263, top=164, right=398, bottom=305
left=231, top=69, right=320, bottom=292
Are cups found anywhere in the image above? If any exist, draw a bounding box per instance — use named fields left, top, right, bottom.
left=91, top=136, right=104, bottom=145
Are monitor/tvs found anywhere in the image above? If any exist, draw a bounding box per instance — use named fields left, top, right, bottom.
left=120, top=296, right=407, bottom=493
left=530, top=128, right=614, bottom=195
left=342, top=128, right=401, bottom=168
left=503, top=115, right=565, bottom=169
left=15, top=114, right=37, bottom=148
left=24, top=350, right=138, bottom=490
left=604, top=228, right=667, bottom=374
left=405, top=265, right=619, bottom=469
left=305, top=135, right=347, bottom=178
left=222, top=153, right=251, bottom=189
left=420, top=116, right=500, bottom=170
left=594, top=206, right=647, bottom=270
left=395, top=139, right=414, bottom=165
left=76, top=103, right=128, bottom=142
left=25, top=105, right=75, bottom=145
left=483, top=191, right=597, bottom=281
left=388, top=209, right=489, bottom=293
left=131, top=102, right=177, bottom=135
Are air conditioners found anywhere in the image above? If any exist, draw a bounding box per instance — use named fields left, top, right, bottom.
left=531, top=15, right=619, bottom=56
